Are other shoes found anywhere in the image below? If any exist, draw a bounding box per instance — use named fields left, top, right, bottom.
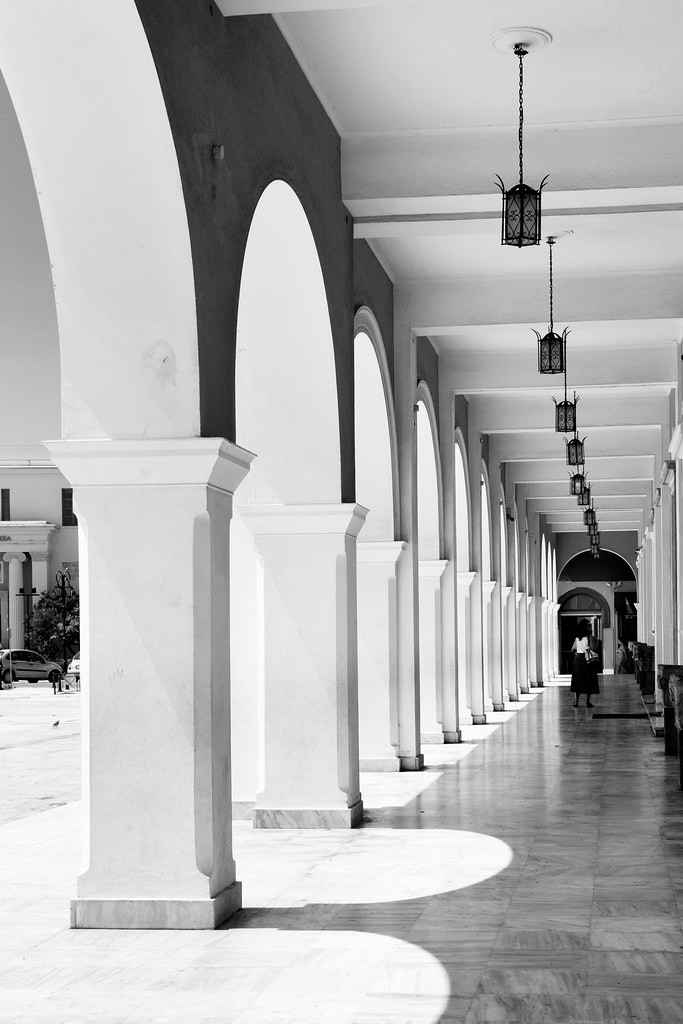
left=573, top=701, right=579, bottom=707
left=585, top=702, right=595, bottom=708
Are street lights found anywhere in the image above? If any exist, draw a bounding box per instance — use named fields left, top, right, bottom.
left=54, top=570, right=75, bottom=673
left=15, top=586, right=39, bottom=650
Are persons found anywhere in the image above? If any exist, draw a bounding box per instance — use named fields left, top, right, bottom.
left=569, top=618, right=600, bottom=707
left=616, top=638, right=631, bottom=674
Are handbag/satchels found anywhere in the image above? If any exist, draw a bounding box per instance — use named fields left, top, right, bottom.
left=585, top=649, right=599, bottom=664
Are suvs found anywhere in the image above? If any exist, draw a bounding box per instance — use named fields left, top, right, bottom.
left=67, top=651, right=80, bottom=676
left=0, top=648, right=65, bottom=684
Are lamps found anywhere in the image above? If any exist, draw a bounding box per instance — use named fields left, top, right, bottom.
left=567, top=431, right=590, bottom=497
left=606, top=581, right=611, bottom=587
left=489, top=26, right=550, bottom=247
left=562, top=391, right=589, bottom=466
left=577, top=466, right=594, bottom=506
left=551, top=330, right=581, bottom=433
left=616, top=581, right=622, bottom=586
left=531, top=231, right=571, bottom=374
left=581, top=499, right=600, bottom=559
